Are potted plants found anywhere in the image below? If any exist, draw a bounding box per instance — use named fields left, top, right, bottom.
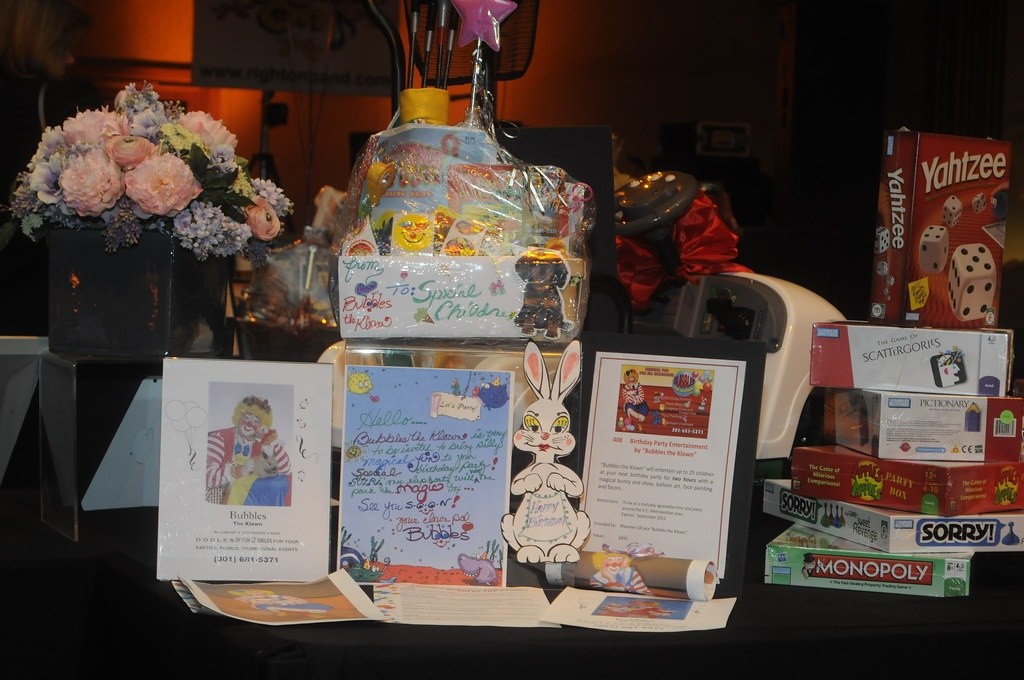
left=6, top=80, right=296, bottom=360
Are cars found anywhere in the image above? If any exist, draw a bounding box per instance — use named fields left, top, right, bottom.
left=610, top=171, right=848, bottom=468
